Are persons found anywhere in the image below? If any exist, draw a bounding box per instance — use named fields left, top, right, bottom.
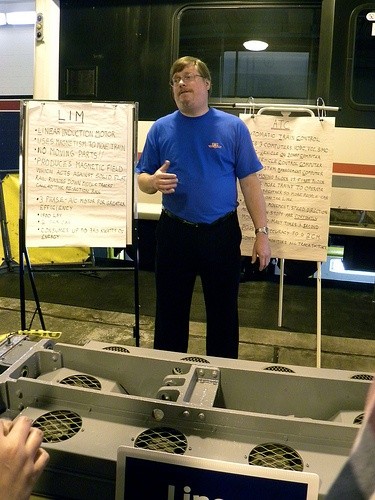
left=139, top=56, right=272, bottom=355
left=1, top=410, right=54, bottom=500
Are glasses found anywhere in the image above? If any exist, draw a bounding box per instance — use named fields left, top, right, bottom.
left=168, top=73, right=205, bottom=88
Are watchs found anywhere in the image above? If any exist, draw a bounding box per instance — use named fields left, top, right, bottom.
left=254, top=226, right=269, bottom=236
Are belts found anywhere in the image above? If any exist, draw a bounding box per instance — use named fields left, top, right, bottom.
left=161, top=207, right=236, bottom=229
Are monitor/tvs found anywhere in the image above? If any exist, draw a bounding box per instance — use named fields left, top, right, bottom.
left=115, top=444, right=319, bottom=499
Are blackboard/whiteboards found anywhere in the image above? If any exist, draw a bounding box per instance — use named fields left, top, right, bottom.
left=1, top=0, right=35, bottom=101
left=20, top=100, right=139, bottom=250
